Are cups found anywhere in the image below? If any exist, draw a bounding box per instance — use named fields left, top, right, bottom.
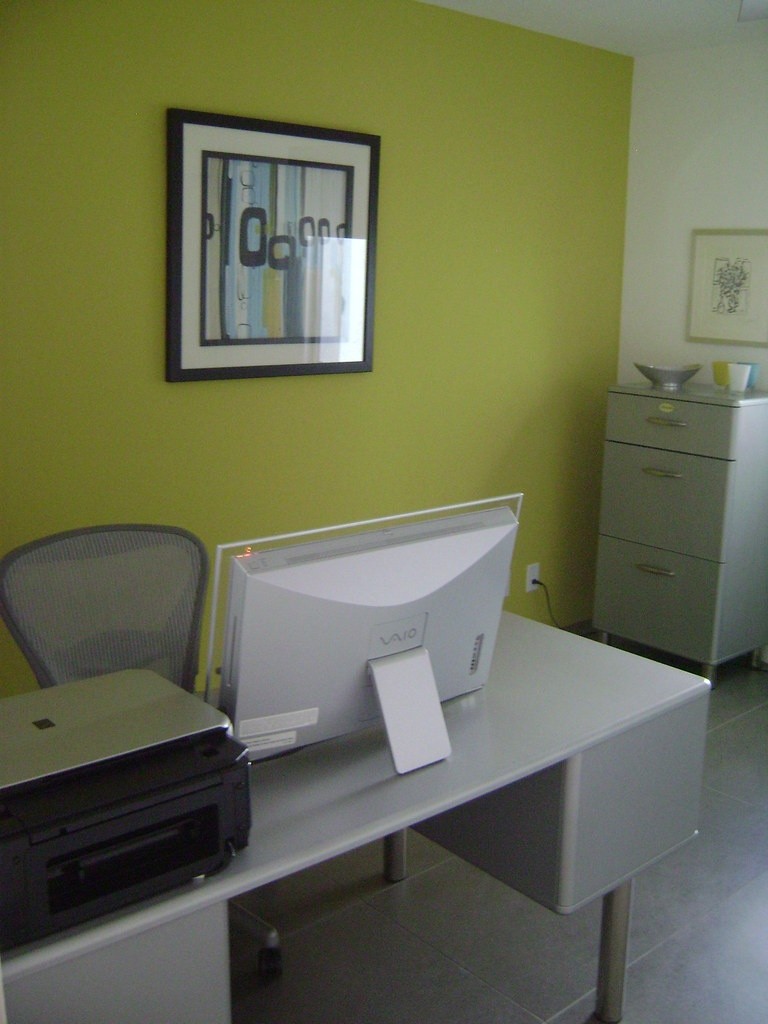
left=712, top=361, right=732, bottom=388
left=727, top=363, right=749, bottom=392
left=737, top=362, right=758, bottom=387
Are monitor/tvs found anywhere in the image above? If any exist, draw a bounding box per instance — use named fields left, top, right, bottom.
left=204, top=490, right=522, bottom=776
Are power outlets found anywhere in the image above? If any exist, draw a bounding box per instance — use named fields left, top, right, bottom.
left=527, top=562, right=539, bottom=592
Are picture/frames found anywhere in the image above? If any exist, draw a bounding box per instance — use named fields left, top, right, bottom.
left=164, top=109, right=382, bottom=385
left=685, top=229, right=768, bottom=349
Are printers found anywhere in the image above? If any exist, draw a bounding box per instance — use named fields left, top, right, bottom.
left=0, top=735, right=252, bottom=951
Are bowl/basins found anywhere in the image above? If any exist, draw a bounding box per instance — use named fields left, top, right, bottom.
left=631, top=361, right=703, bottom=391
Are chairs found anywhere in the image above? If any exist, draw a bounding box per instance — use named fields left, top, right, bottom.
left=0, top=524, right=282, bottom=974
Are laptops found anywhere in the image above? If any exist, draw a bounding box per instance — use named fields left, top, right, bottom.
left=0, top=668, right=233, bottom=797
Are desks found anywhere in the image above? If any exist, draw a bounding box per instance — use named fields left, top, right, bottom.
left=0, top=610, right=713, bottom=1024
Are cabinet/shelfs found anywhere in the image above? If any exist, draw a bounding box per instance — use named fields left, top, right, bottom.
left=594, top=382, right=768, bottom=689
